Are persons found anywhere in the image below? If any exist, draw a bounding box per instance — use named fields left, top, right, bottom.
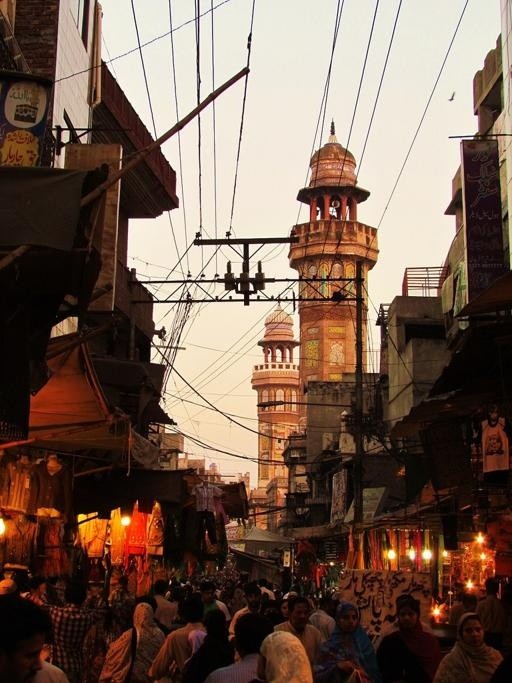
left=147, top=502, right=165, bottom=547
left=0, top=546, right=511, bottom=682
left=0, top=454, right=68, bottom=578
left=190, top=480, right=223, bottom=544
